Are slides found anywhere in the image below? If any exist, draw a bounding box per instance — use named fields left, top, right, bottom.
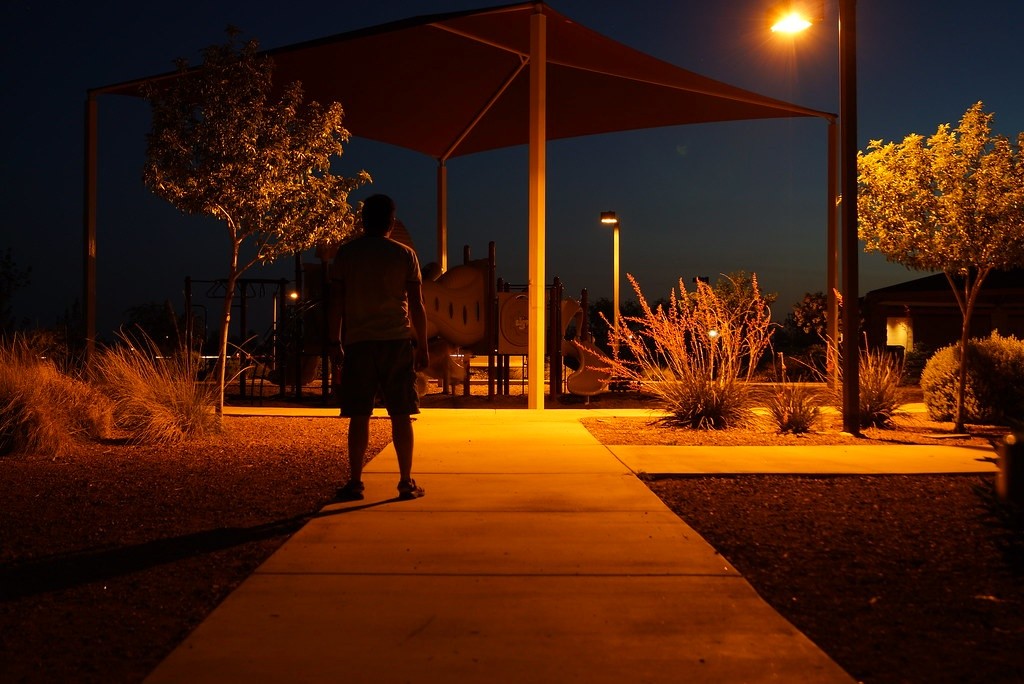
left=422, top=354, right=471, bottom=386
left=566, top=339, right=613, bottom=397
left=266, top=356, right=321, bottom=385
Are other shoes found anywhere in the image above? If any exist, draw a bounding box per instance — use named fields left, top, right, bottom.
left=398, top=478, right=425, bottom=499
left=335, top=478, right=364, bottom=500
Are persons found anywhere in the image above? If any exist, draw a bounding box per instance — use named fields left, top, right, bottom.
left=328, top=194, right=429, bottom=499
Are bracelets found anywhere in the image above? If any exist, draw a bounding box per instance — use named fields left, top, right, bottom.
left=330, top=343, right=340, bottom=347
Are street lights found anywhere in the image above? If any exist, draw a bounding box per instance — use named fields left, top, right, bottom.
left=601, top=212, right=620, bottom=378
left=770, top=0, right=861, bottom=432
